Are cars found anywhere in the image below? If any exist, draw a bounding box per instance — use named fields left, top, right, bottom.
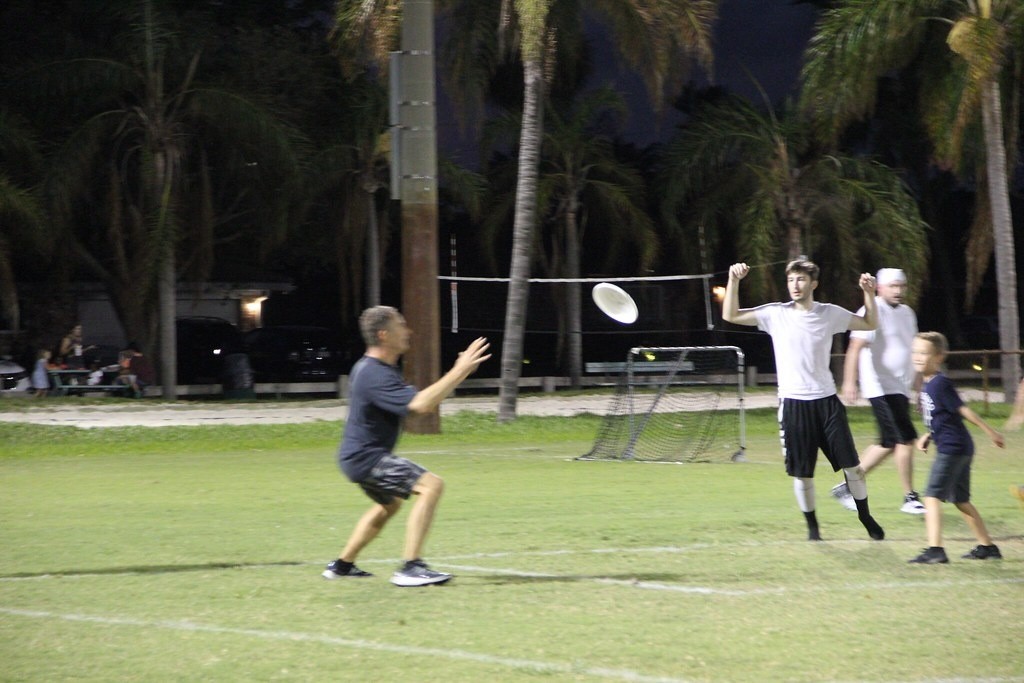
left=0, top=355, right=31, bottom=398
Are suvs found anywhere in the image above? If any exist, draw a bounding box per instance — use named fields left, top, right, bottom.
left=176, top=315, right=341, bottom=401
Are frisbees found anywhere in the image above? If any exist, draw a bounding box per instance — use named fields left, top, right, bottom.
left=591, top=282, right=639, bottom=324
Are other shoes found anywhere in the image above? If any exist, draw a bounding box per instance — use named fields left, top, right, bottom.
left=809, top=524, right=820, bottom=540
left=907, top=546, right=948, bottom=564
left=962, top=543, right=1001, bottom=560
left=857, top=511, right=884, bottom=539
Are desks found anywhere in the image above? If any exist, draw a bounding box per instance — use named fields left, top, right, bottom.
left=46, top=369, right=94, bottom=391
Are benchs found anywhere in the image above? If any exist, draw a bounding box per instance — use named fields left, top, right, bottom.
left=584, top=361, right=695, bottom=378
left=58, top=384, right=133, bottom=399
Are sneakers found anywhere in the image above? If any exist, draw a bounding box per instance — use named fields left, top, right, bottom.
left=390, top=557, right=453, bottom=586
left=834, top=484, right=858, bottom=511
left=900, top=491, right=926, bottom=513
left=322, top=558, right=373, bottom=580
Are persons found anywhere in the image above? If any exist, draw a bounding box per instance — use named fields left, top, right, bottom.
left=905, top=332, right=1007, bottom=565
left=320, top=305, right=492, bottom=587
left=30, top=349, right=59, bottom=400
left=60, top=323, right=104, bottom=396
left=721, top=258, right=885, bottom=542
left=830, top=267, right=927, bottom=514
